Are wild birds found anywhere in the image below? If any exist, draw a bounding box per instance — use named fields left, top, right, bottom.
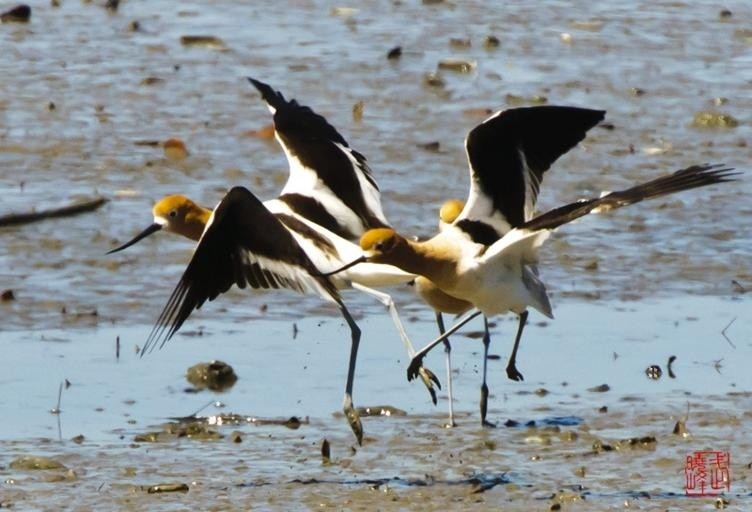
left=105, top=74, right=747, bottom=444
